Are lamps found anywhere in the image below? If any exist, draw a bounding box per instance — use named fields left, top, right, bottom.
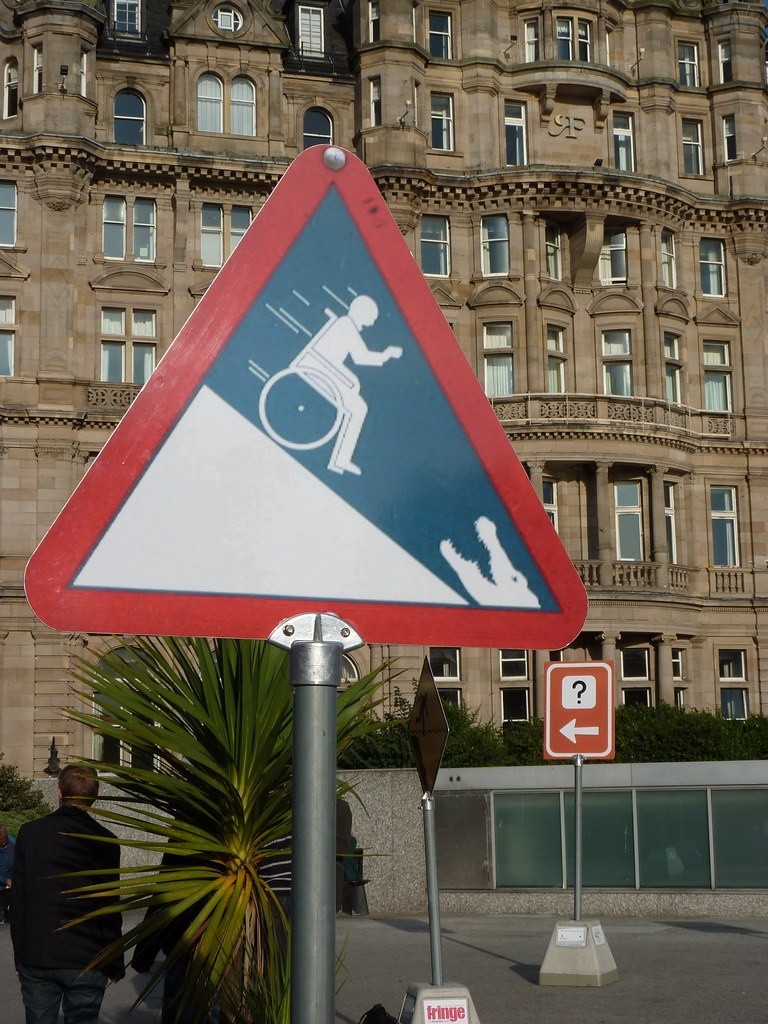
left=396, top=100, right=412, bottom=123
left=500, top=34, right=516, bottom=55
left=593, top=158, right=603, bottom=166
left=57, top=64, right=69, bottom=90
left=628, top=48, right=645, bottom=71
left=750, top=137, right=767, bottom=158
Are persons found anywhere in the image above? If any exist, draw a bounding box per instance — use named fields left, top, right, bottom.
left=131, top=818, right=197, bottom=1024
left=10, top=762, right=126, bottom=1024
left=0, top=828, right=15, bottom=924
